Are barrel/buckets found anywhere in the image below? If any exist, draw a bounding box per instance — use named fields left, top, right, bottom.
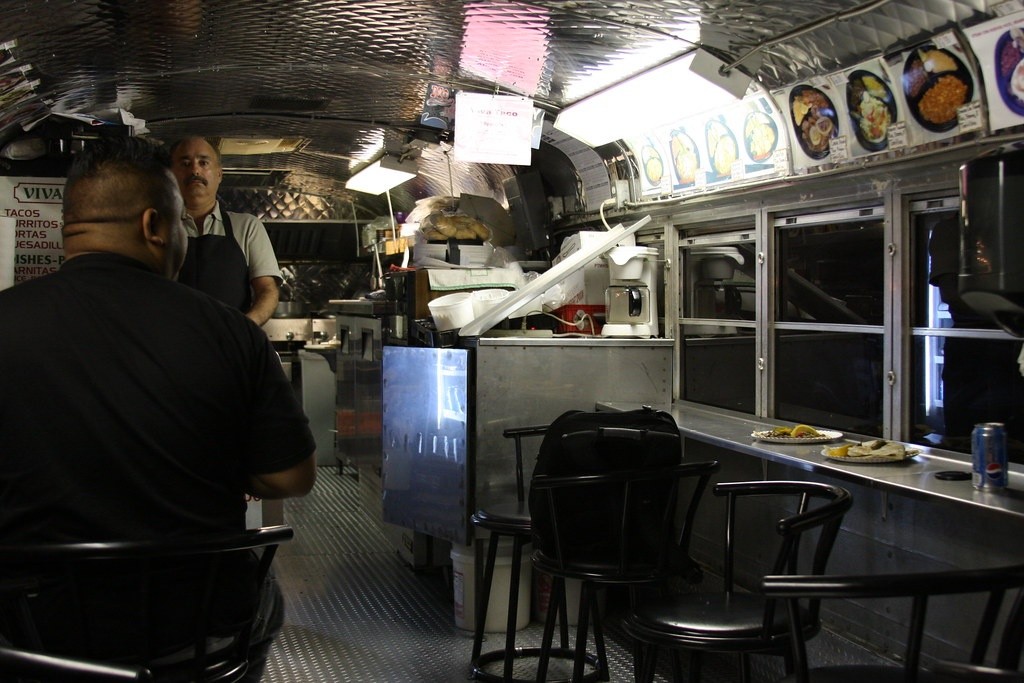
left=472, top=288, right=510, bottom=318
left=428, top=291, right=475, bottom=348
left=449, top=524, right=533, bottom=633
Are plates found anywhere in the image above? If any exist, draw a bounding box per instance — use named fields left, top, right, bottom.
left=902, top=44, right=975, bottom=131
left=705, top=119, right=739, bottom=175
left=749, top=429, right=844, bottom=443
left=743, top=111, right=778, bottom=164
left=845, top=70, right=899, bottom=152
left=789, top=84, right=839, bottom=161
left=668, top=130, right=700, bottom=182
left=993, top=27, right=1024, bottom=116
left=820, top=444, right=923, bottom=463
left=642, top=144, right=664, bottom=186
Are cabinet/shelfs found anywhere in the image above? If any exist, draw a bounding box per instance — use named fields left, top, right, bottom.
left=379, top=336, right=675, bottom=638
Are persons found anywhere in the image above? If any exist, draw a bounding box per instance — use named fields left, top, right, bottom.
left=928, top=213, right=1024, bottom=436
left=0, top=134, right=318, bottom=683
left=169, top=135, right=283, bottom=327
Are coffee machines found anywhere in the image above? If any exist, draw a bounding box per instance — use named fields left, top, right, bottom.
left=599, top=245, right=660, bottom=339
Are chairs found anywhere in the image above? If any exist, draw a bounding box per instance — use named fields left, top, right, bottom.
left=458, top=411, right=1024, bottom=683
left=0, top=523, right=296, bottom=683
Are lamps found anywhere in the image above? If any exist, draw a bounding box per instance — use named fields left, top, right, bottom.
left=344, top=152, right=418, bottom=196
left=554, top=47, right=755, bottom=149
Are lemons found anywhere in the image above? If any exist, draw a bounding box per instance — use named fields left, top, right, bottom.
left=828, top=445, right=852, bottom=457
left=790, top=424, right=819, bottom=437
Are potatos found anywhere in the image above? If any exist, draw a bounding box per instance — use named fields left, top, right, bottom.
left=421, top=212, right=490, bottom=241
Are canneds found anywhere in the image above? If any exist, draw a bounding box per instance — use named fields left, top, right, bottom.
left=971, top=423, right=1008, bottom=492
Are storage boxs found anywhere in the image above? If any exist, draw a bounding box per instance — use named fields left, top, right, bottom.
left=551, top=230, right=636, bottom=306
left=380, top=269, right=513, bottom=319
left=555, top=304, right=607, bottom=335
